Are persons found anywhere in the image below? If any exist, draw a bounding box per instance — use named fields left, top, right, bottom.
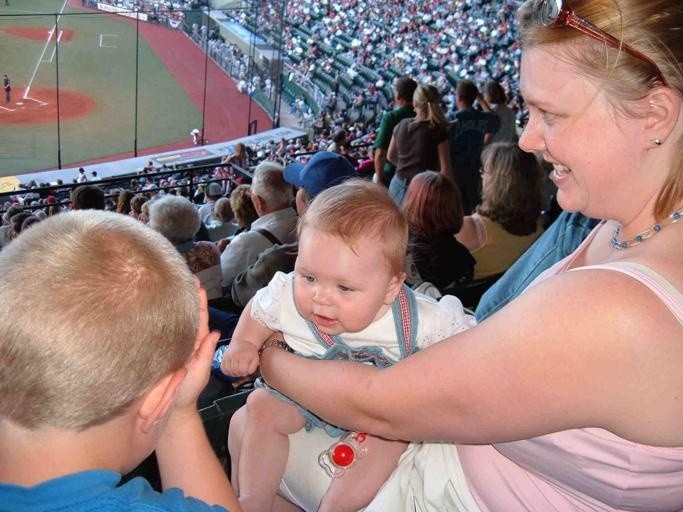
left=2, top=0, right=683, bottom=511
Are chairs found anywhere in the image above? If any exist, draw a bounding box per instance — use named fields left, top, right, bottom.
left=225, top=0, right=466, bottom=106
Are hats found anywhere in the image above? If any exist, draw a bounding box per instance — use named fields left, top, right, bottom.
left=205, top=182, right=223, bottom=197
left=282, top=150, right=359, bottom=200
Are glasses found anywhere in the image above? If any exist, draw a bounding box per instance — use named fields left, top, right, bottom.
left=518, top=0, right=672, bottom=89
